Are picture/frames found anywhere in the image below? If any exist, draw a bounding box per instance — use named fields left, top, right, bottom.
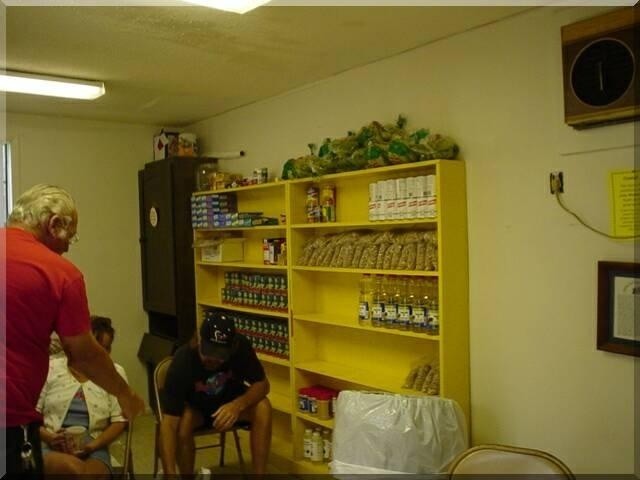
left=0, top=68, right=106, bottom=102
left=595, top=260, right=640, bottom=357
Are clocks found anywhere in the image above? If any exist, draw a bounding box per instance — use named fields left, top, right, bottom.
left=561, top=8, right=639, bottom=127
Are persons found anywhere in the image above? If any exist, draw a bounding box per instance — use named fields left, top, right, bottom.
left=36, top=315, right=128, bottom=480
left=1, top=184, right=142, bottom=475
left=159, top=313, right=272, bottom=475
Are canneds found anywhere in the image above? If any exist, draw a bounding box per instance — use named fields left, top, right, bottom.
left=257, top=168, right=267, bottom=185
left=368, top=175, right=437, bottom=222
left=303, top=183, right=337, bottom=224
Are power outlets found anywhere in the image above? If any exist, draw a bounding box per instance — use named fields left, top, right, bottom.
left=550, top=172, right=564, bottom=194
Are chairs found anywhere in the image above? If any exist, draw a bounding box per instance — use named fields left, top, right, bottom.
left=447, top=446, right=573, bottom=480
left=328, top=389, right=468, bottom=480
left=50, top=407, right=134, bottom=480
left=153, top=356, right=244, bottom=479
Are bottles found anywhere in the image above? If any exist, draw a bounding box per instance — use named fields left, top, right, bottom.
left=299, top=384, right=338, bottom=422
left=303, top=427, right=332, bottom=463
left=357, top=272, right=439, bottom=336
left=305, top=183, right=336, bottom=223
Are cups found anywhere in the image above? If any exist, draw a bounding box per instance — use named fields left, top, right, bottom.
left=61, top=425, right=87, bottom=454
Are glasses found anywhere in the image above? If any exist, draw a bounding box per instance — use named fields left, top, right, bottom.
left=65, top=227, right=80, bottom=244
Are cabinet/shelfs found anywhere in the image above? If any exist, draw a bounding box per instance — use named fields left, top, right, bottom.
left=190, top=158, right=472, bottom=474
left=137, top=157, right=218, bottom=410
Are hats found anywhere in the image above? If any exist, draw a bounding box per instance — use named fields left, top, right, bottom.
left=201, top=314, right=236, bottom=360
